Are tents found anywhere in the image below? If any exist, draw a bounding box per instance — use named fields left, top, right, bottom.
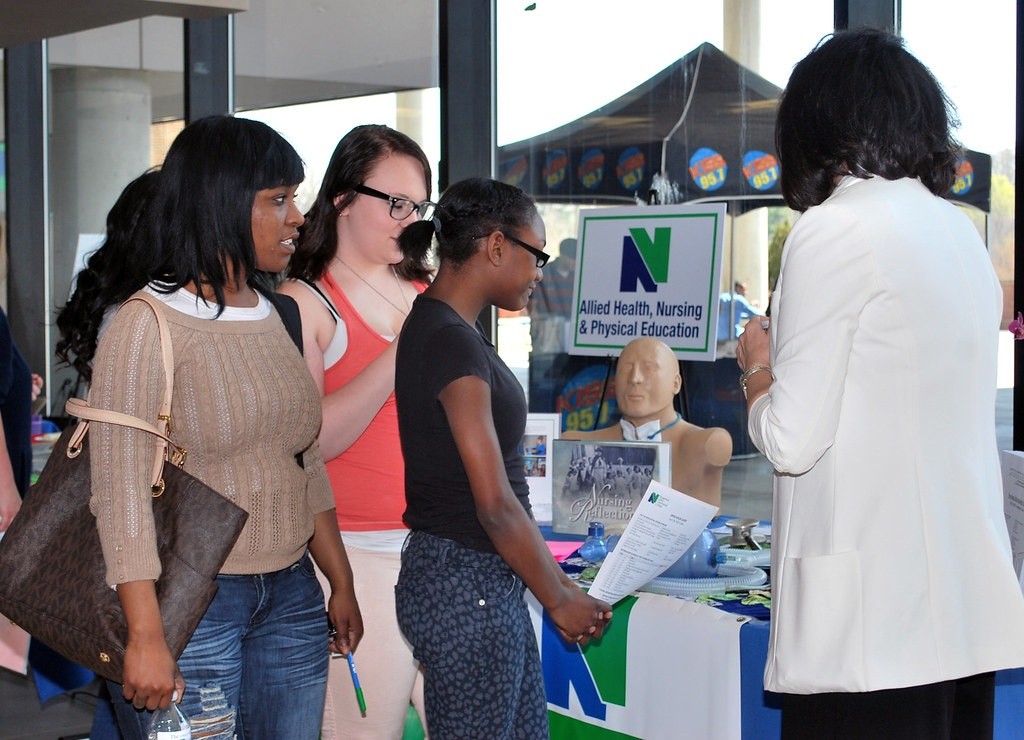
left=435, top=40, right=993, bottom=362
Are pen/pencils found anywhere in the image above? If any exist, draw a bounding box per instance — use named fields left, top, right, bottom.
left=346, top=650, right=367, bottom=718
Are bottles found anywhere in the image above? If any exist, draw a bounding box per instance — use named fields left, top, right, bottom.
left=146, top=691, right=192, bottom=740
left=603, top=527, right=728, bottom=579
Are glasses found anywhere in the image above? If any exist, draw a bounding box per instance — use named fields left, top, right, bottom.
left=353, top=182, right=437, bottom=223
left=471, top=230, right=551, bottom=270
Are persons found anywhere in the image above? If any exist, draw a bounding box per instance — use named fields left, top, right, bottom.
left=276, top=122, right=436, bottom=740
left=736, top=25, right=1024, bottom=739
left=562, top=445, right=652, bottom=503
left=524, top=459, right=546, bottom=477
left=85, top=115, right=364, bottom=740
left=526, top=435, right=548, bottom=456
left=717, top=282, right=769, bottom=362
left=0, top=302, right=44, bottom=539
left=56, top=163, right=175, bottom=396
left=527, top=237, right=580, bottom=354
left=555, top=336, right=734, bottom=521
left=386, top=177, right=611, bottom=740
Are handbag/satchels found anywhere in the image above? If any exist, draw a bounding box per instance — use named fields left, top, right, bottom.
left=1, top=416, right=250, bottom=689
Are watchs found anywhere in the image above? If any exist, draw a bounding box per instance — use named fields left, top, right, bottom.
left=738, top=362, right=775, bottom=401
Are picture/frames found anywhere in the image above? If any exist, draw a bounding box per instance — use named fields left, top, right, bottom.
left=516, top=412, right=562, bottom=526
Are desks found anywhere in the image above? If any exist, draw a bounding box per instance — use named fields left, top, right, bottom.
left=405, top=514, right=1024, bottom=740
